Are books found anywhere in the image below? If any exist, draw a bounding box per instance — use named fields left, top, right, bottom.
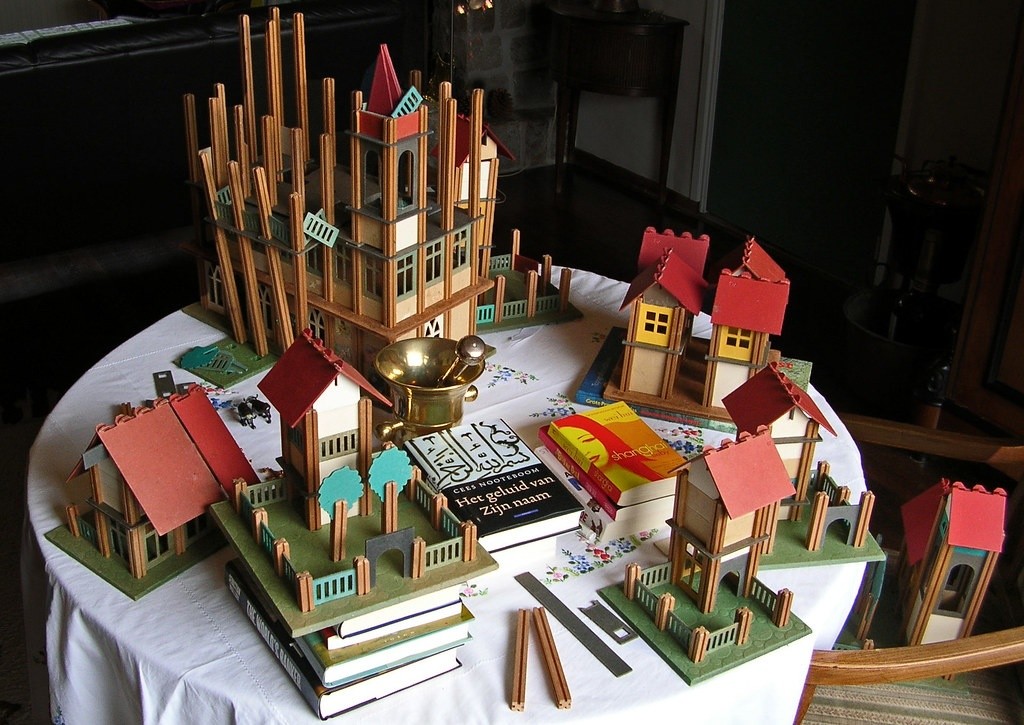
left=224, top=556, right=476, bottom=721
left=401, top=416, right=584, bottom=553
left=535, top=400, right=690, bottom=542
left=575, top=325, right=813, bottom=434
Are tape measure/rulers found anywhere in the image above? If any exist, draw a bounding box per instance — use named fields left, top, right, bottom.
left=514, top=570, right=633, bottom=677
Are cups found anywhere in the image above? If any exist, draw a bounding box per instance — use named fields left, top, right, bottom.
left=376, top=336, right=496, bottom=445
